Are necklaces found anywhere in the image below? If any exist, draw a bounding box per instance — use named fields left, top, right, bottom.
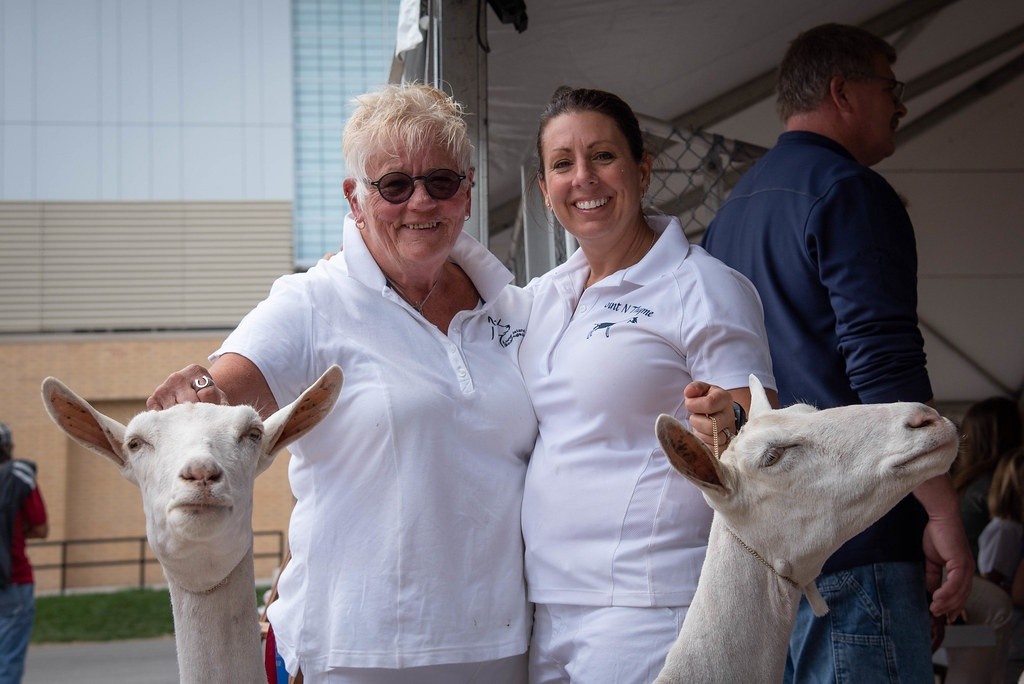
left=648, top=230, right=656, bottom=251
left=386, top=276, right=438, bottom=316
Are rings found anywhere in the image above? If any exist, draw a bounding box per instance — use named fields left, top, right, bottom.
left=193, top=376, right=214, bottom=393
left=723, top=428, right=730, bottom=440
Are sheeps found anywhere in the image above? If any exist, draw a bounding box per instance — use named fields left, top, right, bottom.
left=39, top=363, right=344, bottom=683
left=651, top=373, right=960, bottom=684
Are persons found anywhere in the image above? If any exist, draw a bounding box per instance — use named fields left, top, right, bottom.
left=702, top=23, right=974, bottom=684
left=321, top=84, right=779, bottom=684
left=146, top=83, right=538, bottom=684
left=929, top=380, right=1024, bottom=684
left=0, top=422, right=48, bottom=684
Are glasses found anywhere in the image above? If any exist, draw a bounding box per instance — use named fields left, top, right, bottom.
left=364, top=168, right=466, bottom=204
left=844, top=69, right=906, bottom=105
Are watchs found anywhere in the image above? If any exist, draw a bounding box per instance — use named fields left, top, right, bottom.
left=733, top=401, right=746, bottom=435
